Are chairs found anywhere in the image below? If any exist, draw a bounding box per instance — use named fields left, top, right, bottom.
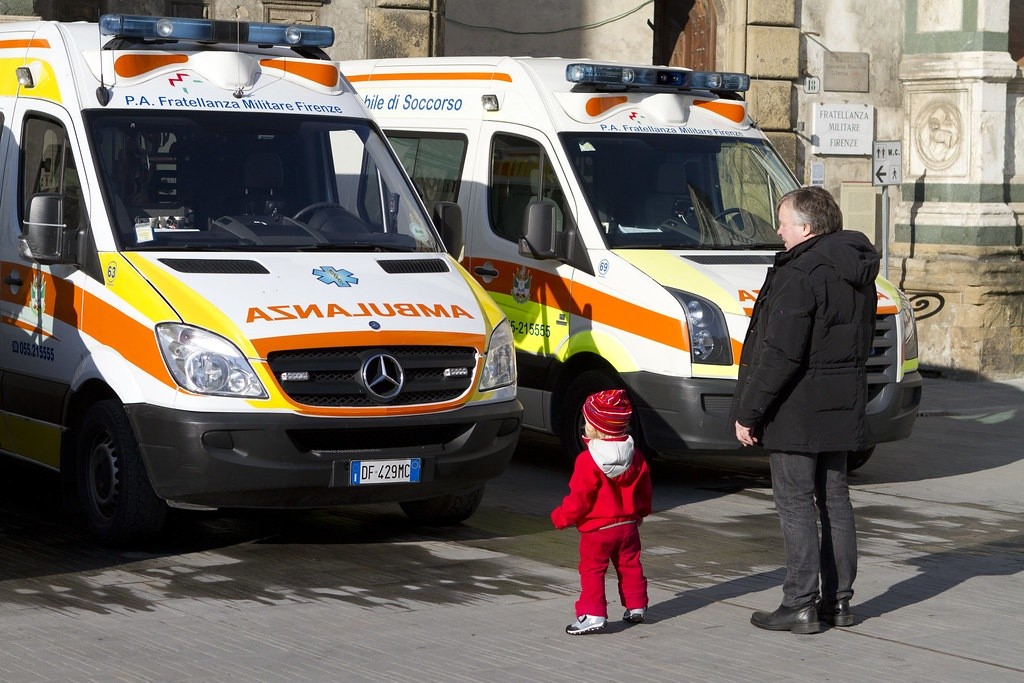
left=637, top=160, right=711, bottom=242
left=526, top=167, right=565, bottom=235
left=243, top=153, right=291, bottom=218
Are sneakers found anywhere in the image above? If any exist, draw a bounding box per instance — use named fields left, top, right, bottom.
left=623, top=609, right=646, bottom=622
left=566, top=614, right=607, bottom=635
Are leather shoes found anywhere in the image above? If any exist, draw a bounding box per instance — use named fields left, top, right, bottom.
left=750, top=600, right=821, bottom=634
left=817, top=598, right=853, bottom=625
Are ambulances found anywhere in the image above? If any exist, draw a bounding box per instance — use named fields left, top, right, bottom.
left=0, top=12, right=524, bottom=553
left=335, top=55, right=921, bottom=490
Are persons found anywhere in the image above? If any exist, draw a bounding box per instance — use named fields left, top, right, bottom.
left=725, top=186, right=880, bottom=635
left=550, top=389, right=652, bottom=635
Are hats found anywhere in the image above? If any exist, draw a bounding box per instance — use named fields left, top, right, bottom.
left=583, top=389, right=632, bottom=436
